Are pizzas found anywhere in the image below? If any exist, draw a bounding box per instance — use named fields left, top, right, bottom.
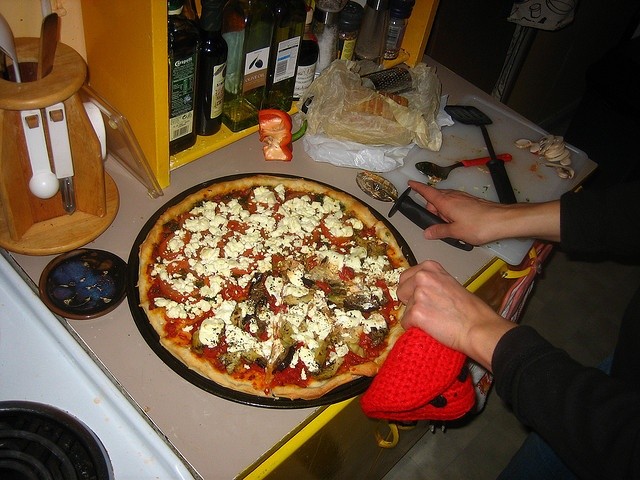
left=136, top=175, right=411, bottom=400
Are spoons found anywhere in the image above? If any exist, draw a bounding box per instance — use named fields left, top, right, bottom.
left=415, top=154, right=512, bottom=181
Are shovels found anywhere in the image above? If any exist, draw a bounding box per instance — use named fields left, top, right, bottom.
left=36, top=13, right=58, bottom=79
left=445, top=105, right=517, bottom=204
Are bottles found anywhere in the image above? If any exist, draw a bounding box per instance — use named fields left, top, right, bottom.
left=167, top=0, right=197, bottom=155
left=243, top=0, right=274, bottom=107
left=337, top=12, right=360, bottom=62
left=197, top=0, right=228, bottom=136
left=313, top=3, right=339, bottom=79
left=353, top=0, right=391, bottom=60
left=222, top=0, right=248, bottom=101
left=292, top=6, right=319, bottom=101
left=262, top=2, right=307, bottom=112
left=382, top=0, right=416, bottom=61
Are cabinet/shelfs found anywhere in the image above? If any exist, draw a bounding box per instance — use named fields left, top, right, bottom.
left=80, top=0, right=442, bottom=191
left=236, top=239, right=549, bottom=480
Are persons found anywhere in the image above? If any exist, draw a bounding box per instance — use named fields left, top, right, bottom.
left=396, top=138, right=640, bottom=479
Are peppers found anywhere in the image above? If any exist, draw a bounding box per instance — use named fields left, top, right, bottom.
left=256, top=107, right=309, bottom=163
